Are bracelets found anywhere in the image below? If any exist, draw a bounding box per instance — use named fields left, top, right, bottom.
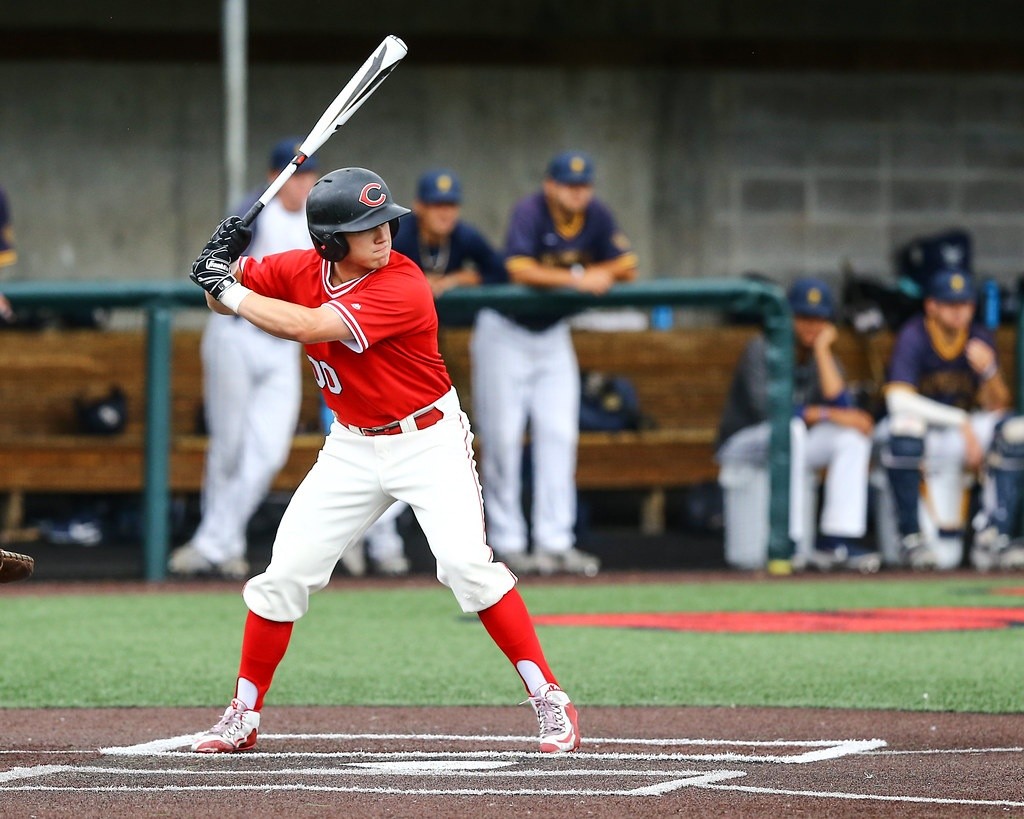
left=981, top=361, right=999, bottom=381
left=818, top=406, right=829, bottom=420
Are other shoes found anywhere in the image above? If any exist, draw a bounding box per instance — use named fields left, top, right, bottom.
left=494, top=545, right=601, bottom=576
left=807, top=530, right=1024, bottom=576
left=166, top=540, right=251, bottom=583
left=339, top=539, right=411, bottom=578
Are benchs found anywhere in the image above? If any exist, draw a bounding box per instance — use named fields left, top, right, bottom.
left=0, top=319, right=1014, bottom=491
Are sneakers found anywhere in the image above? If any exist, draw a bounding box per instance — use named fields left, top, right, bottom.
left=518, top=682, right=581, bottom=753
left=190, top=697, right=261, bottom=754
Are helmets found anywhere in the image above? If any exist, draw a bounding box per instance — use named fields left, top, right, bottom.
left=304, top=167, right=413, bottom=263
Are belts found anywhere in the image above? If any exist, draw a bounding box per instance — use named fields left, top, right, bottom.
left=332, top=407, right=444, bottom=437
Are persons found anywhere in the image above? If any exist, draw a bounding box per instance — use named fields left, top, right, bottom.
left=882, top=269, right=1024, bottom=568
left=190, top=167, right=579, bottom=753
left=714, top=279, right=879, bottom=571
left=169, top=138, right=637, bottom=576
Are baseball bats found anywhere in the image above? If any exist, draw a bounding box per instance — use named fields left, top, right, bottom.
left=191, top=32, right=412, bottom=292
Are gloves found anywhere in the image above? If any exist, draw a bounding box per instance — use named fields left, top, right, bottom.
left=189, top=215, right=252, bottom=300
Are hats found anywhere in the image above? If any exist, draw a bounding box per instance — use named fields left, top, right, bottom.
left=270, top=135, right=320, bottom=174
left=925, top=264, right=976, bottom=304
left=786, top=277, right=836, bottom=318
left=547, top=148, right=595, bottom=186
left=415, top=168, right=462, bottom=204
